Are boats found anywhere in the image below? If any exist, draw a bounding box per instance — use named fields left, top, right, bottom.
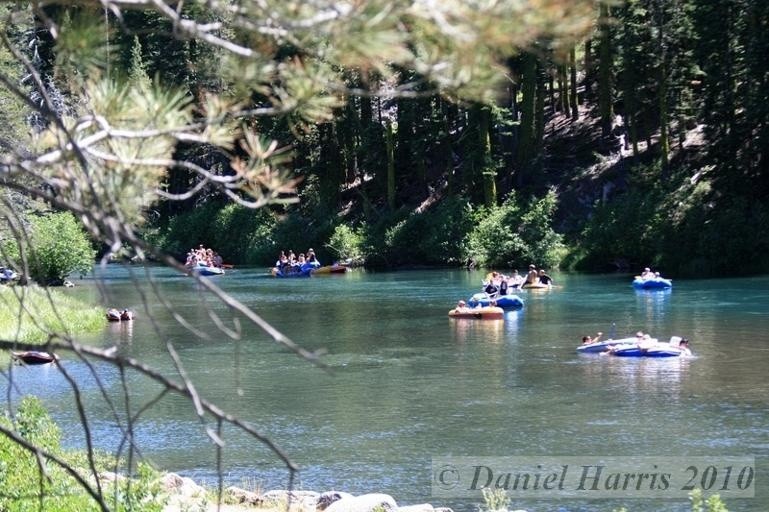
left=608, top=341, right=683, bottom=357
left=271, top=264, right=347, bottom=277
left=14, top=352, right=55, bottom=365
left=104, top=309, right=134, bottom=321
left=482, top=281, right=521, bottom=288
left=467, top=289, right=526, bottom=309
left=0, top=267, right=17, bottom=283
left=189, top=267, right=226, bottom=275
left=573, top=336, right=659, bottom=353
left=515, top=282, right=547, bottom=289
left=634, top=288, right=673, bottom=296
left=446, top=306, right=505, bottom=320
left=629, top=277, right=674, bottom=289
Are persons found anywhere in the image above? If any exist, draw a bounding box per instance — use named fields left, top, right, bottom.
left=578, top=330, right=689, bottom=350
left=634, top=267, right=661, bottom=282
left=121, top=308, right=129, bottom=319
left=275, top=247, right=315, bottom=268
left=454, top=264, right=553, bottom=314
left=183, top=244, right=222, bottom=270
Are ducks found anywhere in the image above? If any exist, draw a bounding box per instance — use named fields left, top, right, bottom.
left=120, top=308, right=132, bottom=321
left=10, top=348, right=60, bottom=365
left=105, top=307, right=119, bottom=321
